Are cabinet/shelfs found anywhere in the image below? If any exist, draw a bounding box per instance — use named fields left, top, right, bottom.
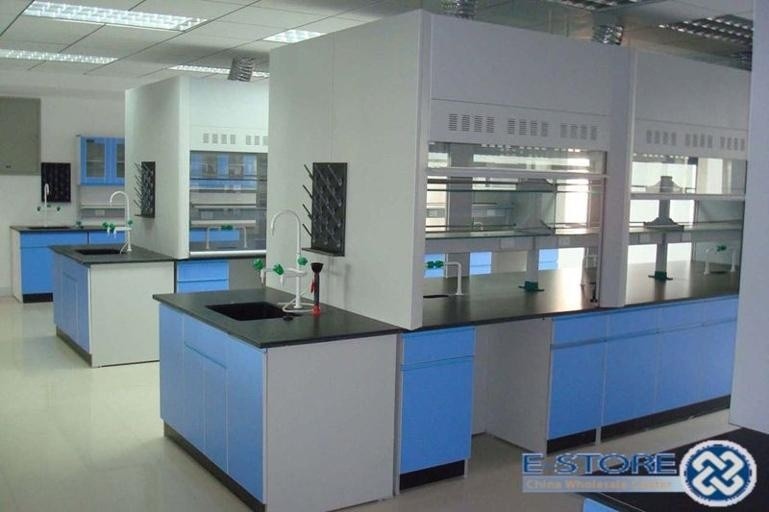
left=627, top=147, right=742, bottom=242
left=77, top=132, right=128, bottom=213
left=189, top=153, right=260, bottom=193
left=579, top=496, right=615, bottom=511
left=399, top=288, right=739, bottom=495
left=11, top=223, right=397, bottom=512
left=420, top=141, right=608, bottom=253
left=424, top=250, right=560, bottom=277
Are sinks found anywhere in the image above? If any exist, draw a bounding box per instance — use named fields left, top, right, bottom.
left=709, top=271, right=728, bottom=274
left=75, top=248, right=128, bottom=256
left=26, top=226, right=71, bottom=230
left=423, top=294, right=448, bottom=298
left=204, top=300, right=302, bottom=322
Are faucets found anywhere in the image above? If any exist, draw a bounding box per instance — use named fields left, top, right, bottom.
left=108, top=191, right=133, bottom=252
left=42, top=183, right=49, bottom=226
left=269, top=208, right=303, bottom=309
